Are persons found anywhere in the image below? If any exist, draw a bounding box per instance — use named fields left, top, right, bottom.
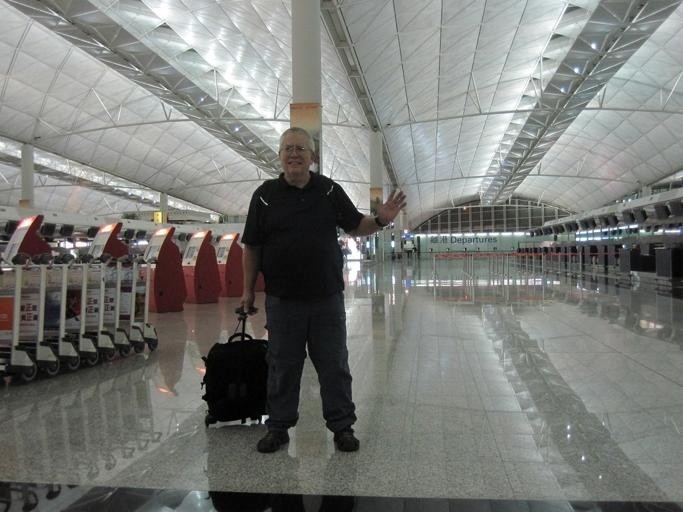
left=412, top=245, right=417, bottom=253
left=240, top=127, right=407, bottom=453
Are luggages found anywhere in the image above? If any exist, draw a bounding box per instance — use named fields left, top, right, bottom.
left=202, top=306, right=268, bottom=427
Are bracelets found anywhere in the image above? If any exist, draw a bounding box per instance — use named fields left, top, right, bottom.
left=372, top=211, right=390, bottom=230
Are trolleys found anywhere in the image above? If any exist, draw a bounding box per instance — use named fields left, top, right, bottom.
left=0, top=250, right=157, bottom=383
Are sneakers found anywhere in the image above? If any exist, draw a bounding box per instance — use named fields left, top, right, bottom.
left=334, top=425, right=359, bottom=451
left=258, top=429, right=289, bottom=453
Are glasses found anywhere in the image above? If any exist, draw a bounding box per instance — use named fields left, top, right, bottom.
left=279, top=146, right=311, bottom=153
